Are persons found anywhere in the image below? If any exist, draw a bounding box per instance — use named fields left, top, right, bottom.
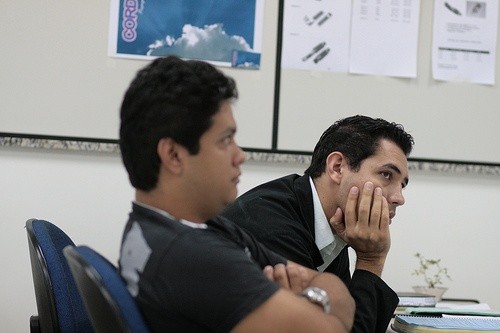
left=117, top=55, right=356, bottom=333
left=221, top=116, right=414, bottom=333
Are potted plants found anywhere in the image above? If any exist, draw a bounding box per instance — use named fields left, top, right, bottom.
left=411, top=253, right=452, bottom=303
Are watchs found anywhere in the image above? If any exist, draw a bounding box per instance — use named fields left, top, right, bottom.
left=299, top=287, right=330, bottom=314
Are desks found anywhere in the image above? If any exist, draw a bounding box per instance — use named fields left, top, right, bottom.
left=384, top=299, right=500, bottom=333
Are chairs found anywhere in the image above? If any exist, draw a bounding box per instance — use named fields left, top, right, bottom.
left=26, top=218, right=95, bottom=333
left=63, top=245, right=147, bottom=333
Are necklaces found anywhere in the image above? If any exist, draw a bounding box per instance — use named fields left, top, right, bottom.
left=134, top=199, right=208, bottom=230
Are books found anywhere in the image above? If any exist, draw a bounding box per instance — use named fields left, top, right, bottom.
left=390, top=293, right=500, bottom=333
